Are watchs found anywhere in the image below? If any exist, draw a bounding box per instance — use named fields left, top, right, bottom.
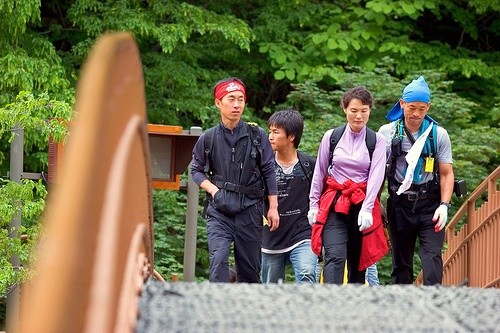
left=440, top=202, right=451, bottom=210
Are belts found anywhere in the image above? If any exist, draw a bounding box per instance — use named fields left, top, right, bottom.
left=397, top=192, right=431, bottom=202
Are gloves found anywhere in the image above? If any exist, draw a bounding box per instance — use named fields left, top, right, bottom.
left=307, top=209, right=318, bottom=226
left=432, top=204, right=447, bottom=232
left=358, top=210, right=373, bottom=231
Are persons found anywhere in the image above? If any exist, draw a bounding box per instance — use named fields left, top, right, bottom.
left=191, top=78, right=279, bottom=284
left=261, top=111, right=319, bottom=285
left=378, top=76, right=454, bottom=286
left=308, top=86, right=389, bottom=285
